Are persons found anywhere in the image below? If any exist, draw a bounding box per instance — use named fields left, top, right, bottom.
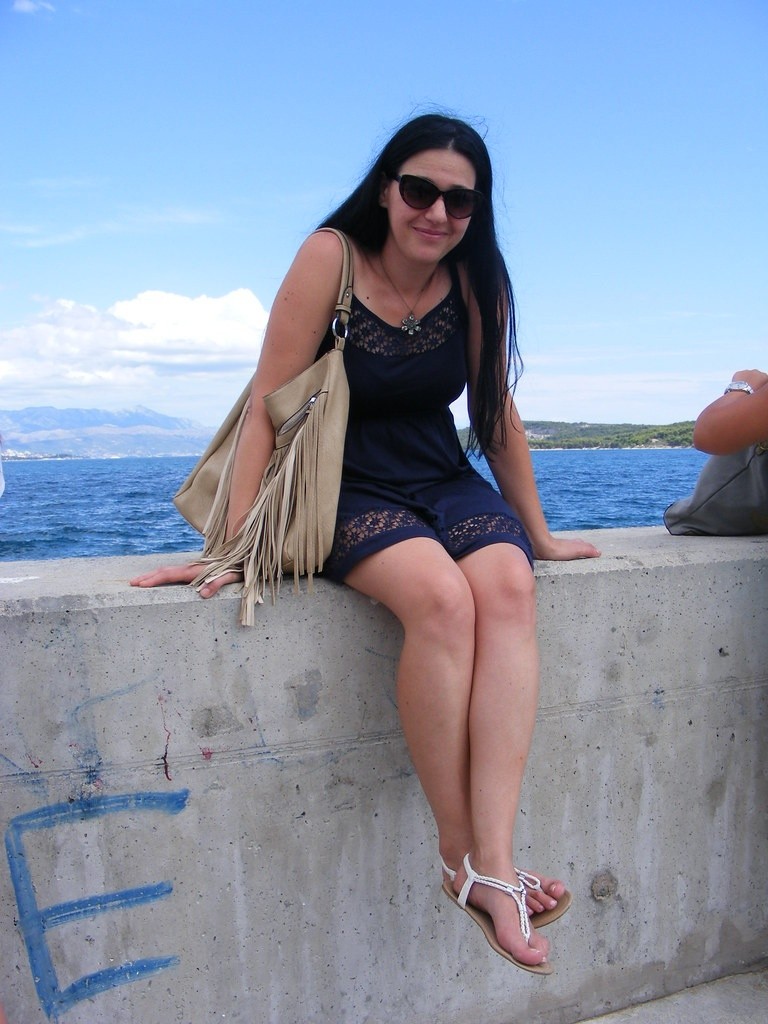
left=693, top=368, right=768, bottom=456
left=130, top=105, right=604, bottom=976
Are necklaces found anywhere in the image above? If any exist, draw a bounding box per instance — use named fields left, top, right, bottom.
left=377, top=253, right=430, bottom=336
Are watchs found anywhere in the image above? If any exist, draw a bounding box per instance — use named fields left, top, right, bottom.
left=724, top=380, right=754, bottom=395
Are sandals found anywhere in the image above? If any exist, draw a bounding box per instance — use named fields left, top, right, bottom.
left=440, top=853, right=573, bottom=928
left=441, top=853, right=553, bottom=976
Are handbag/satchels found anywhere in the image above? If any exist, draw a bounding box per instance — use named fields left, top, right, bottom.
left=662, top=440, right=768, bottom=537
left=172, top=347, right=352, bottom=627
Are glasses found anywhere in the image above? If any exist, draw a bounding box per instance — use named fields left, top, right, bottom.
left=393, top=174, right=485, bottom=220
